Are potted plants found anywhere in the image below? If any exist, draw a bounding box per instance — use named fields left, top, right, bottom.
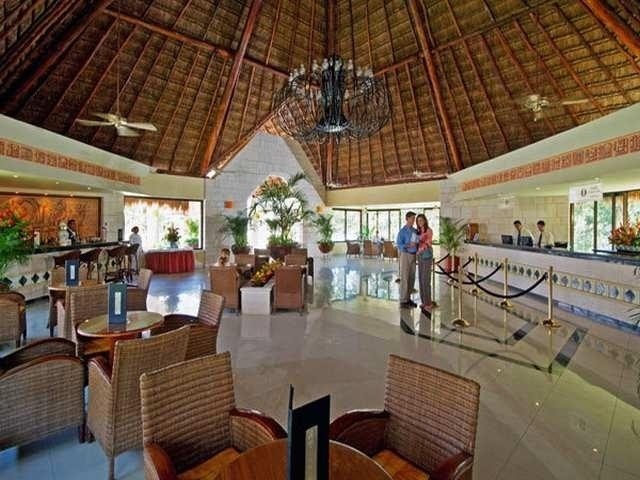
left=219, top=170, right=335, bottom=261
left=433, top=215, right=470, bottom=274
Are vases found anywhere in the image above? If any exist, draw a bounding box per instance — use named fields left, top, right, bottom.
left=618, top=242, right=639, bottom=257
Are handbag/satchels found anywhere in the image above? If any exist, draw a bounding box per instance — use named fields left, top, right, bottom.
left=422, top=247, right=433, bottom=260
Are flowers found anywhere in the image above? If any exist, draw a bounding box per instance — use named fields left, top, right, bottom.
left=0, top=207, right=35, bottom=271
left=608, top=213, right=640, bottom=248
left=161, top=222, right=180, bottom=241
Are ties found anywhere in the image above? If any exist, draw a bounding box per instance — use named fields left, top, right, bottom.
left=517, top=231, right=520, bottom=246
left=538, top=232, right=542, bottom=248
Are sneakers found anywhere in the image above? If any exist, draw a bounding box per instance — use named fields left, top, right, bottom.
left=408, top=301, right=417, bottom=307
left=400, top=303, right=410, bottom=307
left=426, top=305, right=435, bottom=309
left=420, top=304, right=427, bottom=308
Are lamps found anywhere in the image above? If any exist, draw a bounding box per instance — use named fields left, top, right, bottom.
left=270, top=0, right=392, bottom=144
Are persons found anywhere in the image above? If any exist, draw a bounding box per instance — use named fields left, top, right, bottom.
left=395, top=211, right=419, bottom=310
left=212, top=248, right=236, bottom=266
left=534, top=219, right=555, bottom=248
left=415, top=214, right=435, bottom=309
left=67, top=218, right=80, bottom=244
left=376, top=236, right=385, bottom=265
left=511, top=219, right=535, bottom=245
left=373, top=229, right=380, bottom=244
left=129, top=226, right=144, bottom=276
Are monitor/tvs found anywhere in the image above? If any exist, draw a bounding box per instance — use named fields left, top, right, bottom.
left=501, top=235, right=513, bottom=244
left=517, top=236, right=533, bottom=247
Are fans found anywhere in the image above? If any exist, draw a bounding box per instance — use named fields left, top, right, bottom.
left=517, top=0, right=589, bottom=122
left=76, top=0, right=158, bottom=139
